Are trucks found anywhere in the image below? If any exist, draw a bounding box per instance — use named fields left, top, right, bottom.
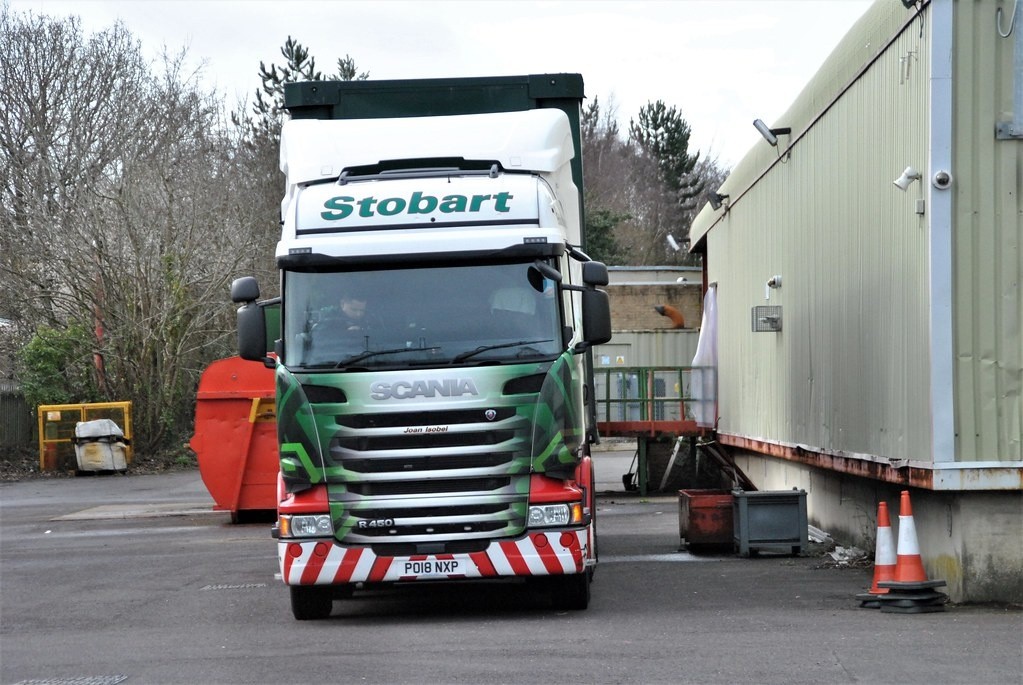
left=270, top=72, right=614, bottom=620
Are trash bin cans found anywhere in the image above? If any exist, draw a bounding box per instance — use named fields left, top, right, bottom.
left=73, top=418, right=130, bottom=477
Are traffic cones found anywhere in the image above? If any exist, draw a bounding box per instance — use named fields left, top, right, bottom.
left=854, top=502, right=899, bottom=610
left=878, top=491, right=950, bottom=613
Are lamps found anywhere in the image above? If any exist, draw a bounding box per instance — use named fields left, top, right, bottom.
left=893, top=166, right=926, bottom=214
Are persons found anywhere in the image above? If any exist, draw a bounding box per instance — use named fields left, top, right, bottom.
left=313, top=288, right=389, bottom=334
